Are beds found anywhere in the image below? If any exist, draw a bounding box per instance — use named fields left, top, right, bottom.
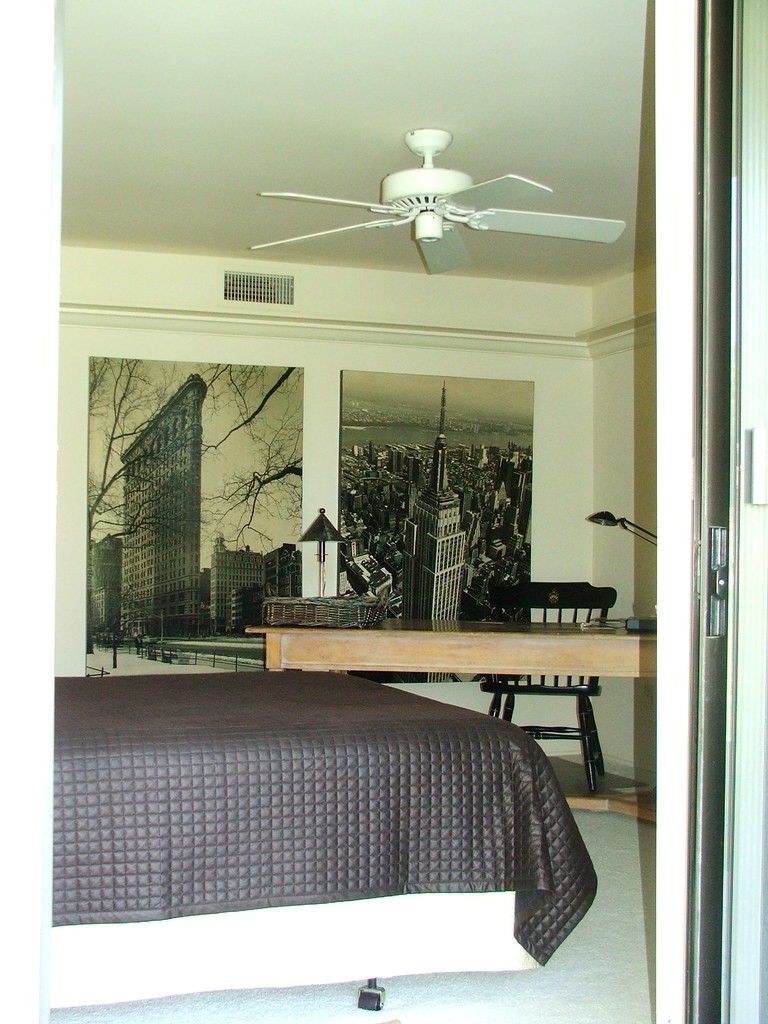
left=54, top=673, right=600, bottom=1011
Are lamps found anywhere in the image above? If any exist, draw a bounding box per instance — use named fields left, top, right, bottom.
left=296, top=508, right=344, bottom=599
left=587, top=511, right=657, bottom=545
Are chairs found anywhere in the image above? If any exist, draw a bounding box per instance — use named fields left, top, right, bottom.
left=479, top=582, right=617, bottom=791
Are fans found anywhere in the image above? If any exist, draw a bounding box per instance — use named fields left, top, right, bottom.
left=248, top=130, right=628, bottom=251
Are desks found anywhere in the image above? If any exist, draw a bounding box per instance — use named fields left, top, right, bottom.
left=245, top=621, right=658, bottom=825
